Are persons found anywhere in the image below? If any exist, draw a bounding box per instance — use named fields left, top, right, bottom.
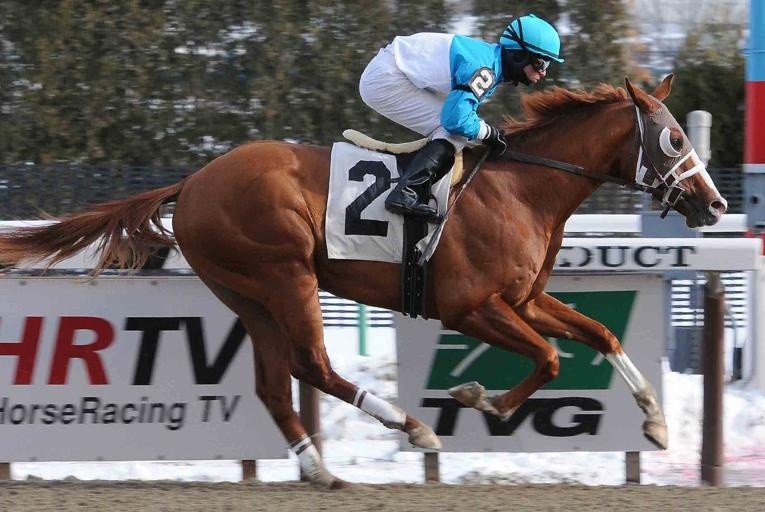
left=358, top=13, right=564, bottom=223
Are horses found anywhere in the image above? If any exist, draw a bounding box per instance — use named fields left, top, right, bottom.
left=0, top=73, right=727, bottom=489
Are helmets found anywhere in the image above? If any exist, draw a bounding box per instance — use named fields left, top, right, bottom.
left=499, top=14, right=564, bottom=63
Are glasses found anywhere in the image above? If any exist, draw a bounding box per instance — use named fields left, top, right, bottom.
left=529, top=54, right=550, bottom=72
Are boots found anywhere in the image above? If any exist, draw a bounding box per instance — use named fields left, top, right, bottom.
left=385, top=139, right=454, bottom=224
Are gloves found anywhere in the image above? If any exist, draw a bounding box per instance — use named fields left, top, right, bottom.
left=484, top=123, right=509, bottom=159
